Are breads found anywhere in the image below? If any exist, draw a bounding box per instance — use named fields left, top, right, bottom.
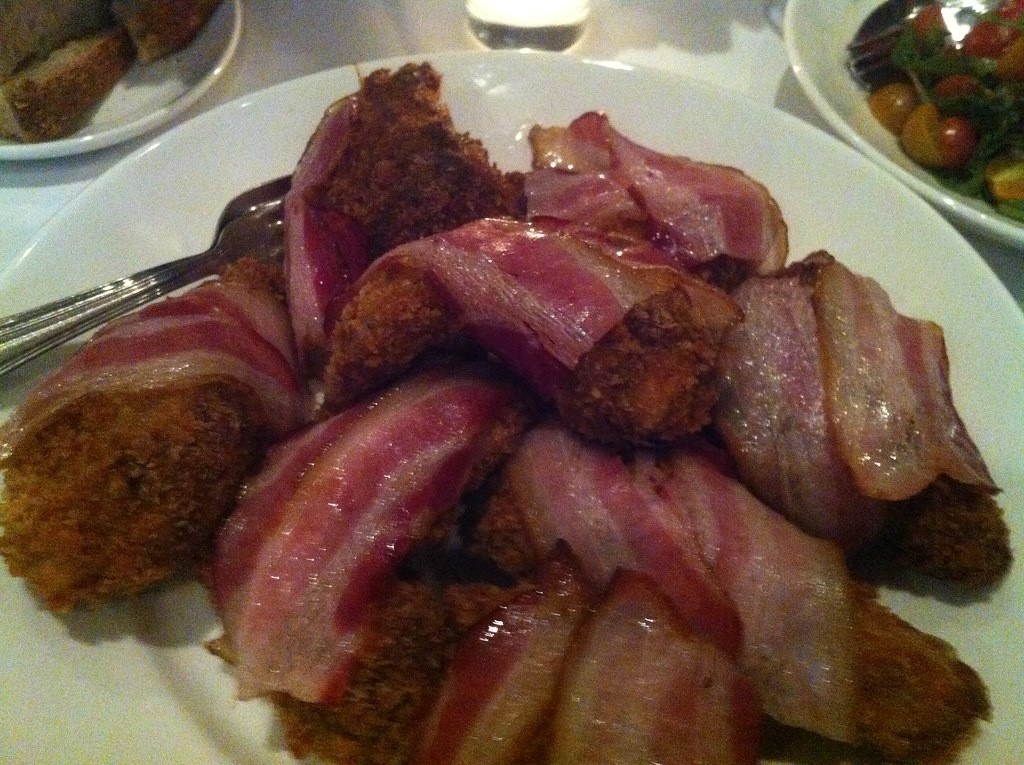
left=0, top=0, right=229, bottom=144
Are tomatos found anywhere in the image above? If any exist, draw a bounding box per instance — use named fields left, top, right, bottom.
left=872, top=0, right=1024, bottom=204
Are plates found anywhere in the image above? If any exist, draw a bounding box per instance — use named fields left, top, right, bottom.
left=784, top=1, right=1024, bottom=247
left=0, top=0, right=244, bottom=160
left=0, top=49, right=1024, bottom=765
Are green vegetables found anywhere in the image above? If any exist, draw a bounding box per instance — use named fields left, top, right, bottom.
left=891, top=11, right=1024, bottom=223
